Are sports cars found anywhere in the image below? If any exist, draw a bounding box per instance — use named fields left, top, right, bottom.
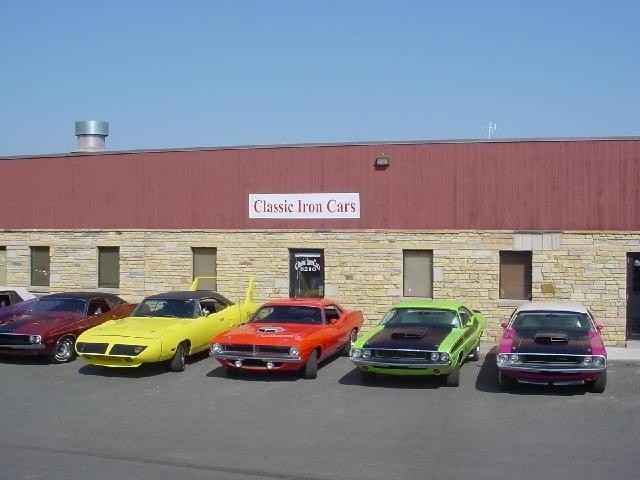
left=496, top=302, right=609, bottom=394
left=0, top=288, right=149, bottom=364
left=350, top=300, right=485, bottom=385
left=209, top=297, right=363, bottom=382
left=75, top=275, right=264, bottom=373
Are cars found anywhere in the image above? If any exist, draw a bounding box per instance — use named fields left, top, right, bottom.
left=0, top=287, right=36, bottom=308
